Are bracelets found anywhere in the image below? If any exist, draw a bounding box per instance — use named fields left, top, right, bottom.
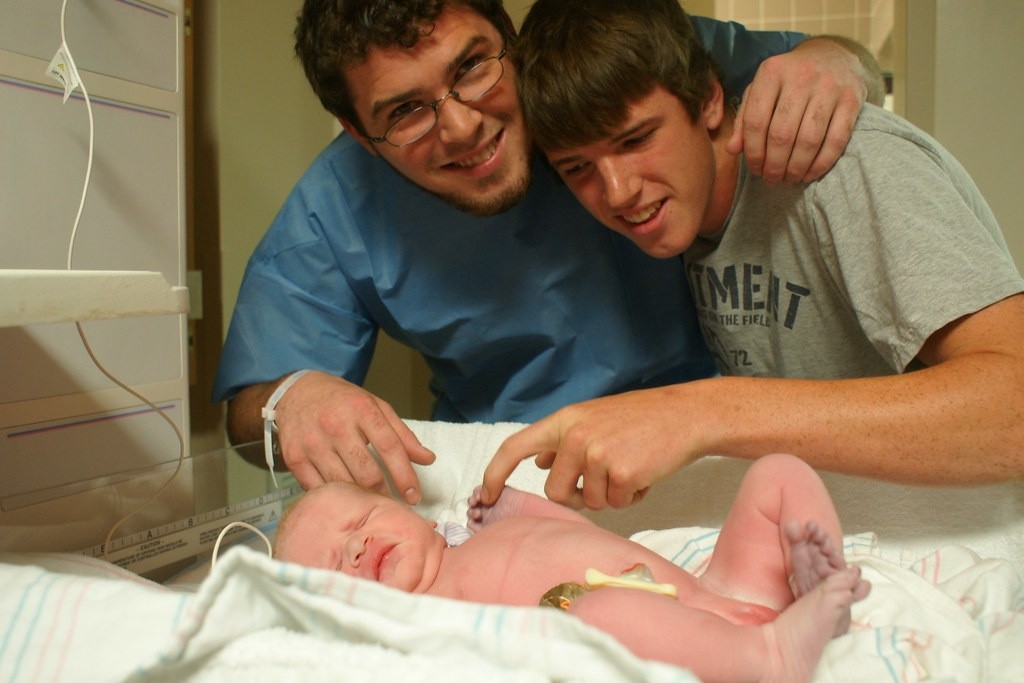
left=260, top=366, right=314, bottom=490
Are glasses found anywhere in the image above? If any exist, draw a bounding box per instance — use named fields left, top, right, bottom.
left=366, top=35, right=511, bottom=148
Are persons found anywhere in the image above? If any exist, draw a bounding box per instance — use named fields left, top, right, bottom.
left=269, top=451, right=873, bottom=683
left=209, top=1, right=889, bottom=509
left=476, top=0, right=1024, bottom=508
